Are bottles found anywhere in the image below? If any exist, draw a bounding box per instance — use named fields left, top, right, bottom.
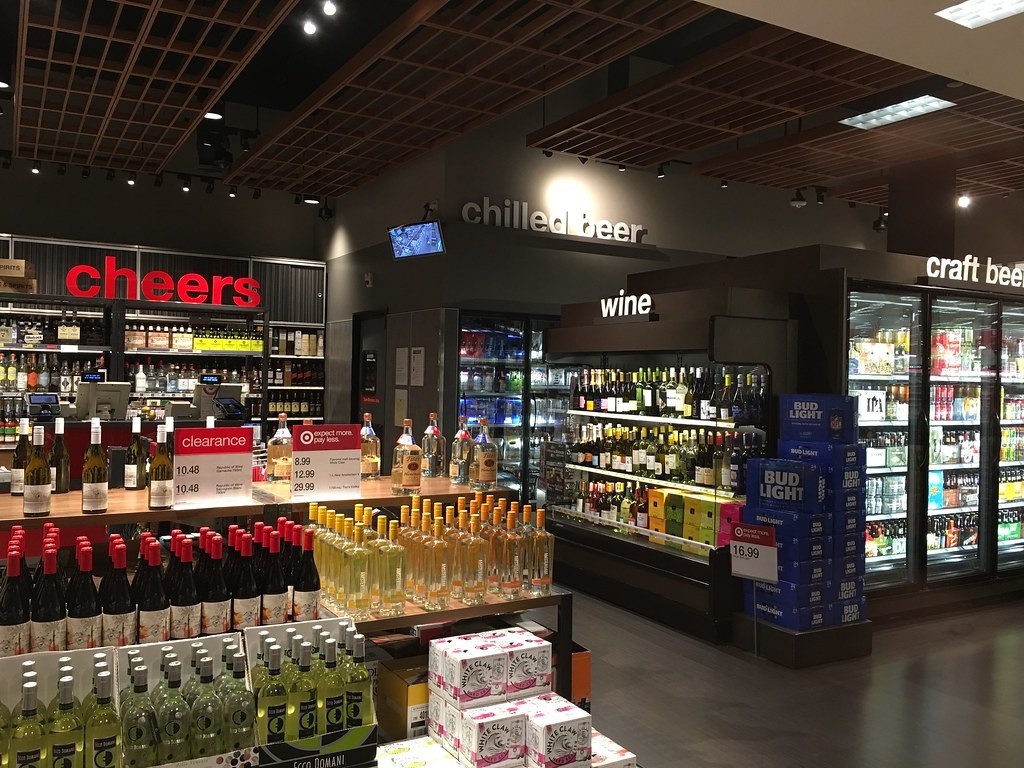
left=23, top=426, right=52, bottom=517
left=123, top=416, right=146, bottom=490
left=49, top=418, right=71, bottom=494
left=0, top=489, right=553, bottom=767
left=573, top=368, right=769, bottom=540
left=267, top=413, right=292, bottom=484
left=82, top=426, right=109, bottom=514
left=83, top=417, right=107, bottom=465
left=0, top=319, right=324, bottom=444
left=10, top=417, right=33, bottom=496
left=422, top=412, right=447, bottom=477
left=391, top=418, right=422, bottom=495
left=849, top=324, right=1024, bottom=557
left=449, top=415, right=473, bottom=484
left=359, top=412, right=380, bottom=480
left=165, top=417, right=174, bottom=465
left=469, top=418, right=498, bottom=490
left=148, top=424, right=174, bottom=510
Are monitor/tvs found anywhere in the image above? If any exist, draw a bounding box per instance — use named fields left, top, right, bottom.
left=386, top=218, right=447, bottom=261
left=193, top=384, right=242, bottom=418
left=75, top=381, right=131, bottom=420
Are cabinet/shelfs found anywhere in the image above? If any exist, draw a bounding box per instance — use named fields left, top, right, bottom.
left=0, top=306, right=325, bottom=428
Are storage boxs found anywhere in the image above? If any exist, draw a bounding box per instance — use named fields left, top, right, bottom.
left=161, top=615, right=636, bottom=768
left=738, top=395, right=869, bottom=629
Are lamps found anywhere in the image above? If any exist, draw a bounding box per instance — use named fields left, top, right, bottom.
left=0, top=95, right=333, bottom=220
left=545, top=151, right=891, bottom=231
